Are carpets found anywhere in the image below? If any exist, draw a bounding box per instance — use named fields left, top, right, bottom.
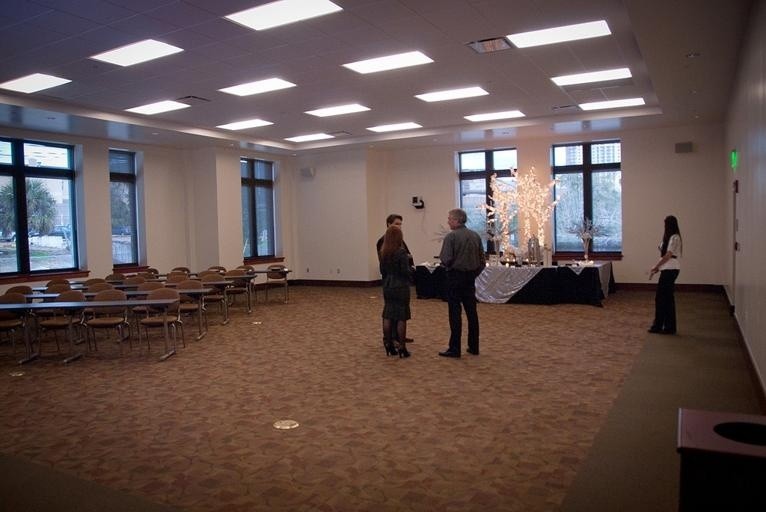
left=559, top=291, right=765, bottom=511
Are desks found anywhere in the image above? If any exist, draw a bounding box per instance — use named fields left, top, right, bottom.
left=415, top=255, right=617, bottom=308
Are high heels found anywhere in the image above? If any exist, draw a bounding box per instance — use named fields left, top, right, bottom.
left=398, top=347, right=411, bottom=359
left=386, top=343, right=398, bottom=357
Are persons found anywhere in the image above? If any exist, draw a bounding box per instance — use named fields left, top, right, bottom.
left=380, top=226, right=412, bottom=358
left=439, top=209, right=486, bottom=358
left=376, top=214, right=416, bottom=349
left=647, top=216, right=682, bottom=334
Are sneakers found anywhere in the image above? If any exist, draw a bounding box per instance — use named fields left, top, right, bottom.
left=438, top=347, right=462, bottom=358
left=395, top=335, right=414, bottom=343
left=466, top=347, right=480, bottom=356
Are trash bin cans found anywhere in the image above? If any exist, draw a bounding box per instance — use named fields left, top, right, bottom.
left=676, top=408, right=766, bottom=512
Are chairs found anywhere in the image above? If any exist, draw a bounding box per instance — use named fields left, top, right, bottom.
left=1, top=264, right=293, bottom=365
